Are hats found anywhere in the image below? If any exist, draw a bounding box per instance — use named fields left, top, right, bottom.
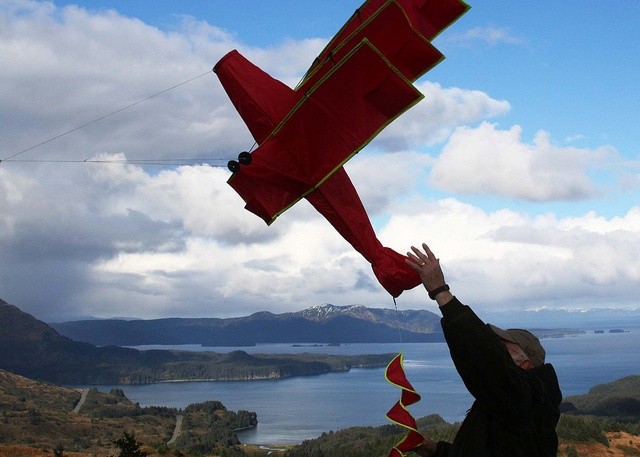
left=486, top=322, right=545, bottom=368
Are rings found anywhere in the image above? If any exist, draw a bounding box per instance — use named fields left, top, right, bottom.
left=421, top=262, right=425, bottom=267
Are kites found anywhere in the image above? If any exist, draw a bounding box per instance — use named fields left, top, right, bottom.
left=211, top=0, right=472, bottom=457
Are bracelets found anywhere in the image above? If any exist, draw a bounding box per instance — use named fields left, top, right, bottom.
left=429, top=285, right=450, bottom=299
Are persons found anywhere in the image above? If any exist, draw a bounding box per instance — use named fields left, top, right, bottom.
left=405, top=246, right=563, bottom=457
